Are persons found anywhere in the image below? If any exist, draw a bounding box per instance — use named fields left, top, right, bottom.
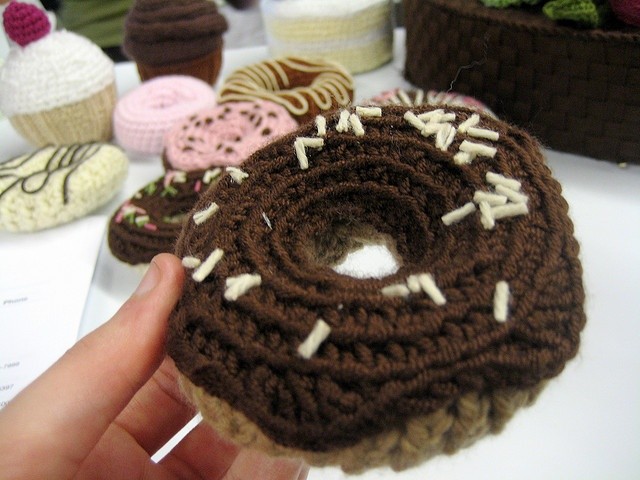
left=0, top=252, right=312, bottom=480
